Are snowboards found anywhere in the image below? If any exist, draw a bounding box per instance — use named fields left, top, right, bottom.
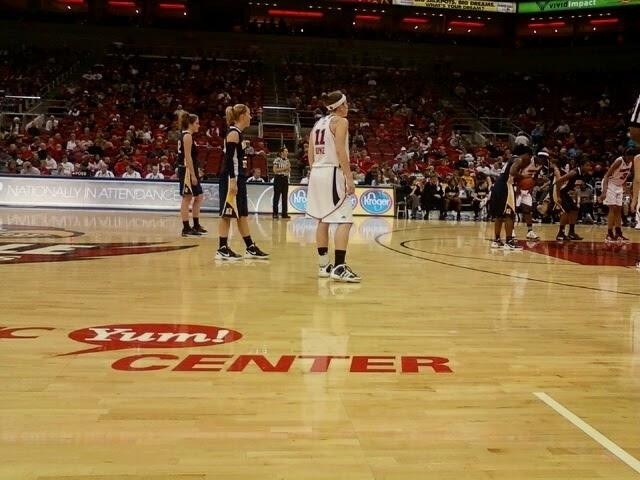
left=517, top=178, right=535, bottom=190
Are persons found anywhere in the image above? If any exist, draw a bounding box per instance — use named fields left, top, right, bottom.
left=303, top=89, right=362, bottom=284
left=487, top=144, right=533, bottom=251
left=508, top=150, right=550, bottom=241
left=174, top=109, right=209, bottom=237
left=553, top=158, right=593, bottom=242
left=271, top=147, right=291, bottom=218
left=214, top=103, right=271, bottom=261
left=598, top=148, right=636, bottom=243
left=630, top=153, right=640, bottom=269
left=2, top=47, right=267, bottom=183
left=277, top=46, right=638, bottom=226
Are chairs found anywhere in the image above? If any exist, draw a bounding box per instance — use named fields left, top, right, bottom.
left=244, top=243, right=270, bottom=259
left=215, top=248, right=242, bottom=261
left=491, top=230, right=523, bottom=251
left=318, top=263, right=334, bottom=278
left=605, top=233, right=630, bottom=243
left=556, top=233, right=571, bottom=240
left=182, top=227, right=201, bottom=237
left=193, top=224, right=208, bottom=235
left=0, top=60, right=268, bottom=187
left=282, top=215, right=291, bottom=219
left=278, top=60, right=633, bottom=184
left=567, top=232, right=583, bottom=240
left=331, top=264, right=361, bottom=283
left=526, top=230, right=540, bottom=241
left=273, top=213, right=278, bottom=218
